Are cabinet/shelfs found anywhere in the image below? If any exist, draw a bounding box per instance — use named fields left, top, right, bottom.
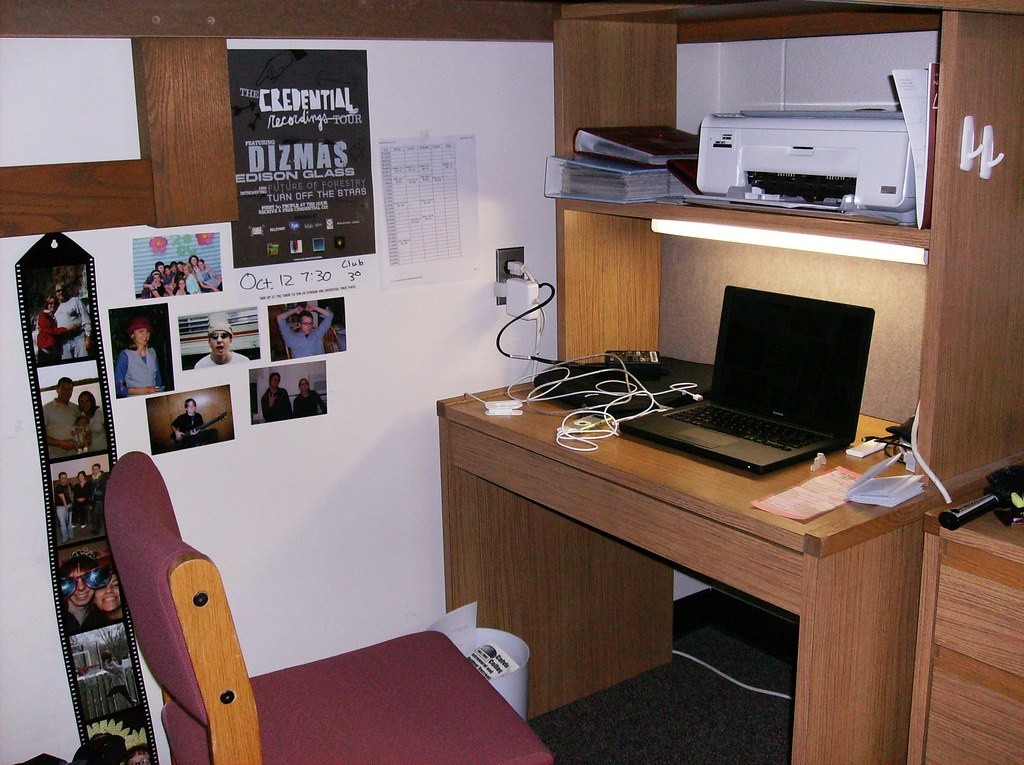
left=906, top=492, right=1024, bottom=765
left=435, top=0, right=1023, bottom=764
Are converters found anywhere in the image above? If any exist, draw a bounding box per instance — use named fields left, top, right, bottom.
left=604, top=350, right=663, bottom=381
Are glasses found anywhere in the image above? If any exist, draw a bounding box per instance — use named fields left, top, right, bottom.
left=300, top=321, right=314, bottom=326
left=48, top=302, right=58, bottom=305
left=209, top=332, right=229, bottom=339
left=56, top=289, right=67, bottom=293
left=61, top=566, right=112, bottom=600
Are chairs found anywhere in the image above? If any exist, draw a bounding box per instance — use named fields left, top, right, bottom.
left=105, top=452, right=554, bottom=765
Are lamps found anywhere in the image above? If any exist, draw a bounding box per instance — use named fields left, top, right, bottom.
left=650, top=219, right=929, bottom=269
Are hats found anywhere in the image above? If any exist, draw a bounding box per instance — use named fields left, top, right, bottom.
left=207, top=322, right=233, bottom=339
left=127, top=318, right=153, bottom=333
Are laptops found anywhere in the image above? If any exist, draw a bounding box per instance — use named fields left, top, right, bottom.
left=620, top=285, right=875, bottom=474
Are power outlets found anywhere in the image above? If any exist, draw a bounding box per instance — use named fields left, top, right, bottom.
left=496, top=247, right=525, bottom=307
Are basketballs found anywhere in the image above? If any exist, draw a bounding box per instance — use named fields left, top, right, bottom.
left=101, top=650, right=112, bottom=660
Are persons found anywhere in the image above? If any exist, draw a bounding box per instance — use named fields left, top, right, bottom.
left=171, top=399, right=219, bottom=450
left=105, top=660, right=136, bottom=707
left=124, top=744, right=150, bottom=765
left=115, top=317, right=164, bottom=396
left=59, top=555, right=123, bottom=632
left=278, top=304, right=334, bottom=358
left=54, top=463, right=110, bottom=543
left=142, top=256, right=223, bottom=299
left=43, top=377, right=108, bottom=459
left=193, top=325, right=251, bottom=370
left=261, top=373, right=327, bottom=421
left=33, top=283, right=92, bottom=362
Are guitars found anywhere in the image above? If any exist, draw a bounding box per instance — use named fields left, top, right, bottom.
left=169, top=410, right=228, bottom=449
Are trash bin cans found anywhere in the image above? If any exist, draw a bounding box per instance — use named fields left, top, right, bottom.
left=446, top=627, right=531, bottom=721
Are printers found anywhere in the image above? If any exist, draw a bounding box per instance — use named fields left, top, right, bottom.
left=684, top=110, right=918, bottom=228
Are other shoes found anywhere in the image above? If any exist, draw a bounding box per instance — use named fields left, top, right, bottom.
left=70, top=534, right=74, bottom=540
left=81, top=524, right=86, bottom=529
left=72, top=523, right=78, bottom=528
left=63, top=537, right=68, bottom=543
left=92, top=528, right=100, bottom=535
